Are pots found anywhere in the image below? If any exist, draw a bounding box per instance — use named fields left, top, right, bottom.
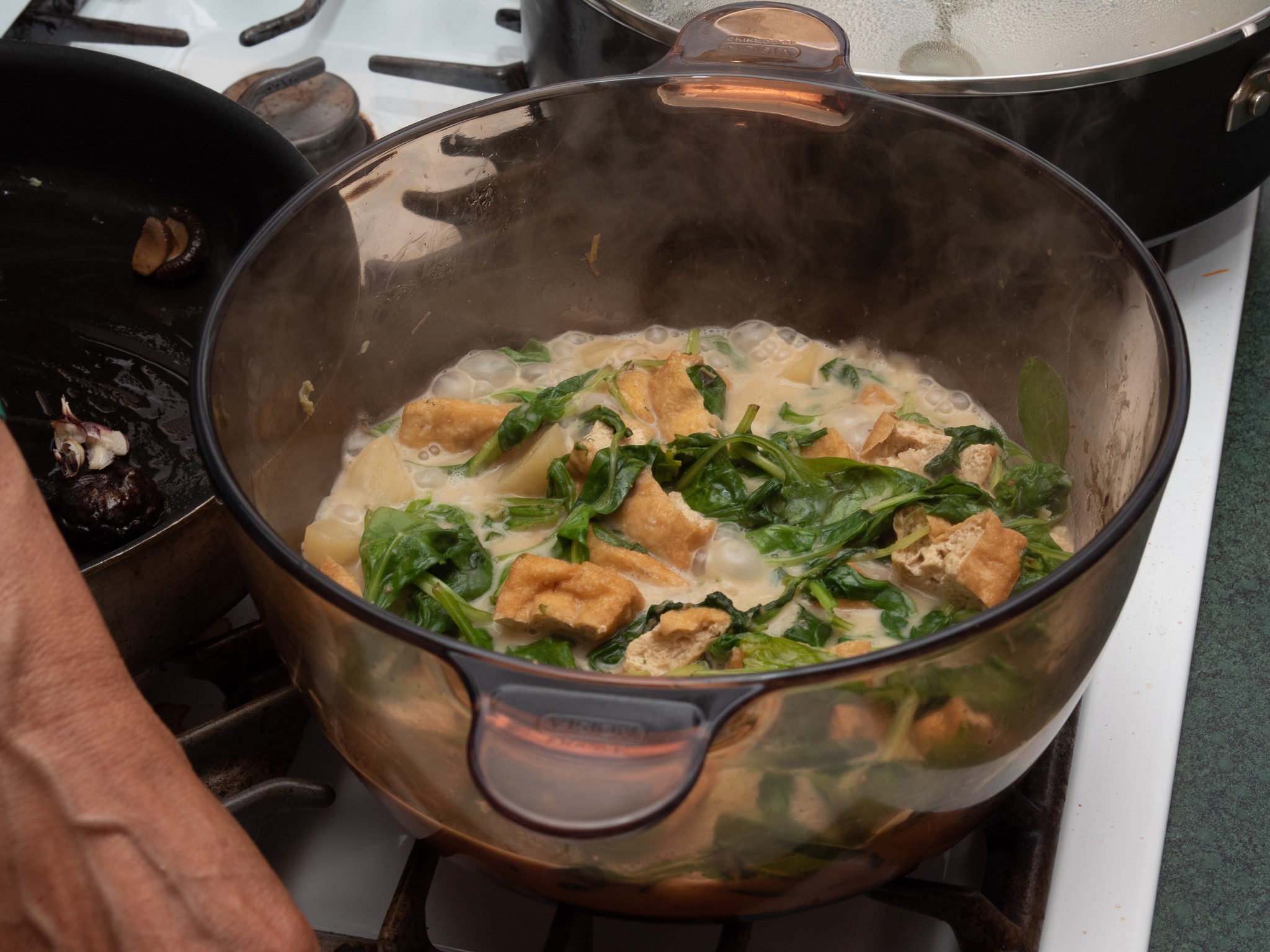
left=189, top=1, right=1193, bottom=922
left=519, top=0, right=1270, bottom=251
left=2, top=41, right=361, bottom=702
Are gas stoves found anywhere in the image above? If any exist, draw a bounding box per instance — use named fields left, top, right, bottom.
left=1, top=0, right=1179, bottom=952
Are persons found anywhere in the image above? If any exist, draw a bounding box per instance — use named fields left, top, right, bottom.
left=0, top=418, right=325, bottom=952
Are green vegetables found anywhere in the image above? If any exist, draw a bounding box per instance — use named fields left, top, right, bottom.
left=301, top=341, right=1079, bottom=897
